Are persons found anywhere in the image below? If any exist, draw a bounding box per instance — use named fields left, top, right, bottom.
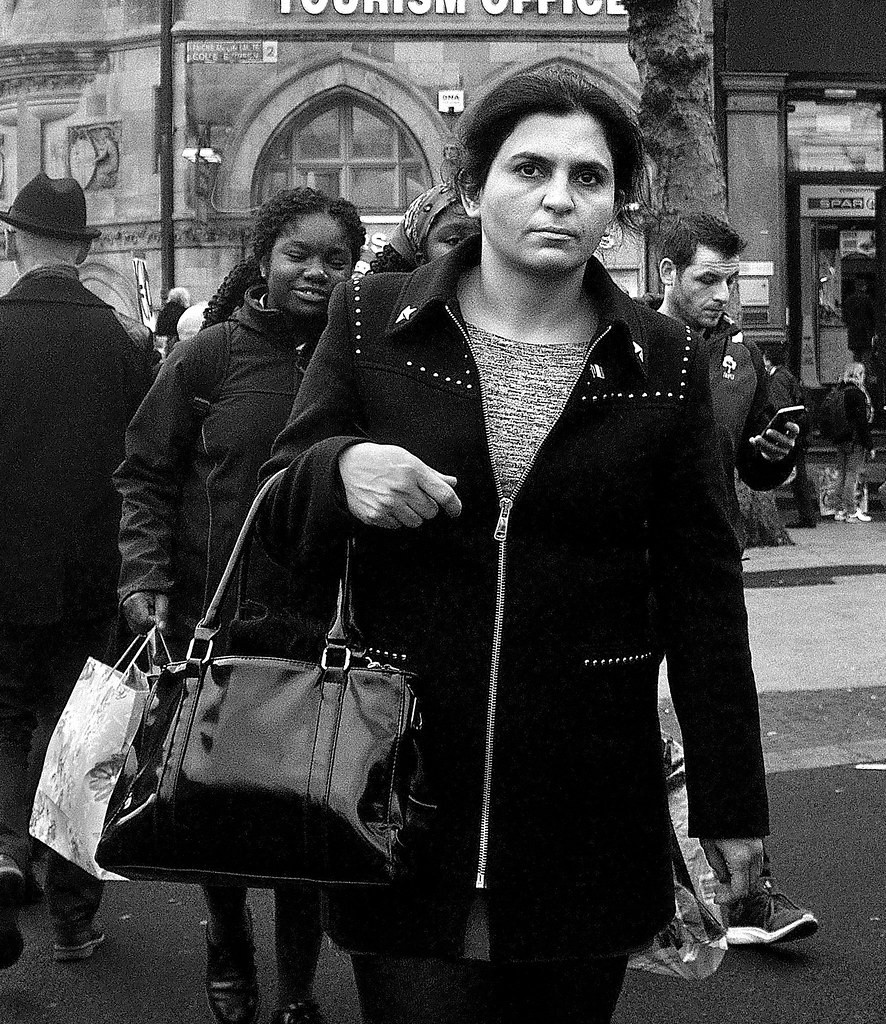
left=841, top=280, right=880, bottom=361
left=0, top=171, right=150, bottom=965
left=247, top=70, right=772, bottom=1024
left=634, top=211, right=820, bottom=943
left=370, top=179, right=479, bottom=271
left=155, top=287, right=210, bottom=338
left=111, top=185, right=369, bottom=1024
left=831, top=362, right=876, bottom=523
left=764, top=346, right=819, bottom=529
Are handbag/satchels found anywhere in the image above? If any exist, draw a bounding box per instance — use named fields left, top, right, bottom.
left=28, top=471, right=438, bottom=895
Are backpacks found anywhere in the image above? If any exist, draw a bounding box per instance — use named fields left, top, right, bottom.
left=820, top=385, right=857, bottom=434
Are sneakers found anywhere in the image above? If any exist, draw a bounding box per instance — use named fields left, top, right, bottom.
left=720, top=874, right=819, bottom=944
left=272, top=999, right=330, bottom=1024
left=201, top=902, right=260, bottom=1024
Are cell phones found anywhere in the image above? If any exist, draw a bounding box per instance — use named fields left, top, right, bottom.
left=762, top=405, right=805, bottom=444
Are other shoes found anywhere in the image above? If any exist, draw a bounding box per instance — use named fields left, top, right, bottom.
left=0, top=853, right=30, bottom=968
left=834, top=509, right=846, bottom=520
left=845, top=510, right=872, bottom=524
left=54, top=926, right=105, bottom=959
left=785, top=519, right=819, bottom=528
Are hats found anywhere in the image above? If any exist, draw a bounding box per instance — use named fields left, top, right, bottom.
left=0, top=172, right=102, bottom=241
